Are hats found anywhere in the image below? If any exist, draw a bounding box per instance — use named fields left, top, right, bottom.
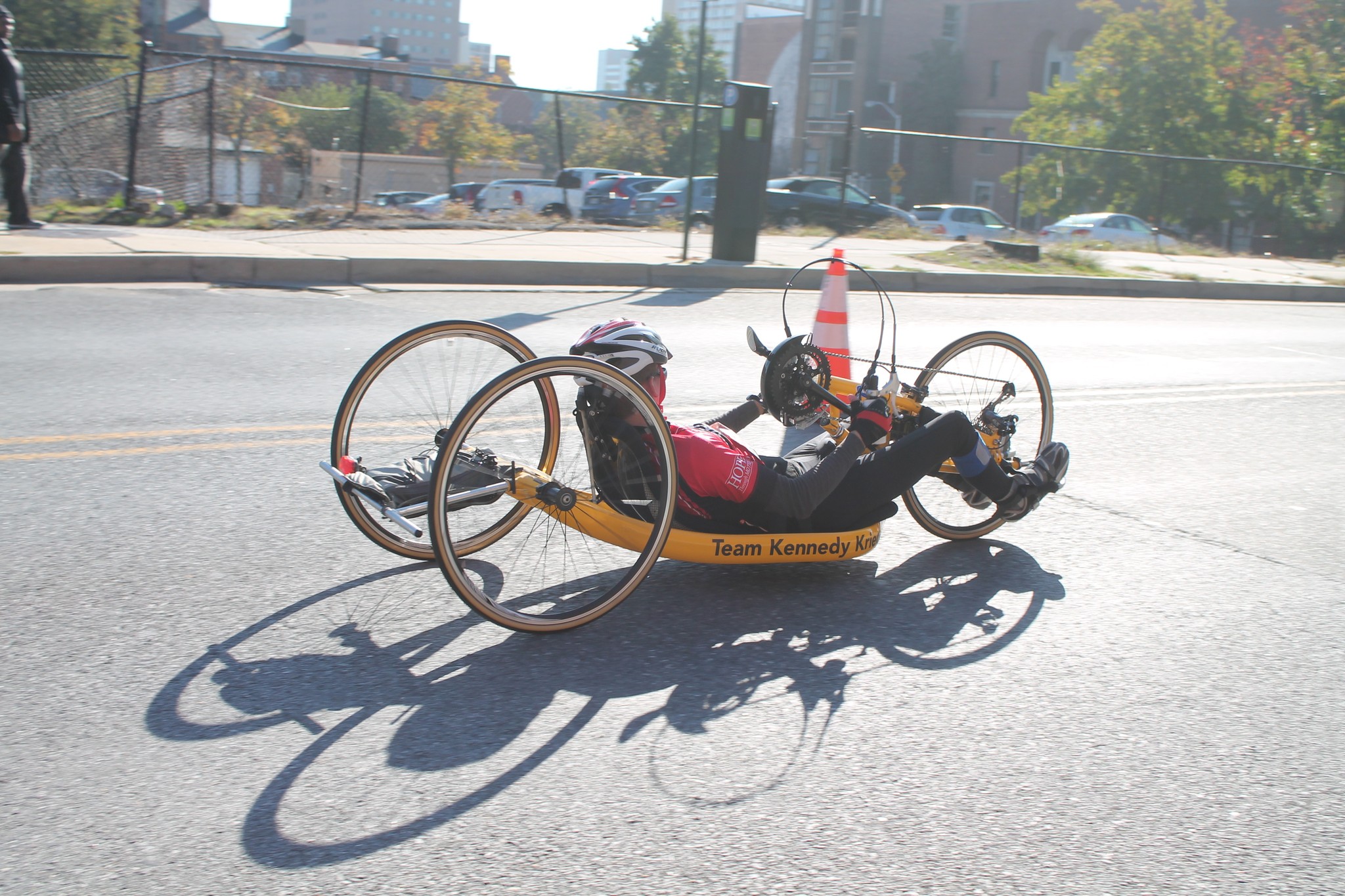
left=0, top=5, right=15, bottom=18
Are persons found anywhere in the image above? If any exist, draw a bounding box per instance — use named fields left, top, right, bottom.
left=571, top=318, right=1071, bottom=531
left=0, top=5, right=46, bottom=230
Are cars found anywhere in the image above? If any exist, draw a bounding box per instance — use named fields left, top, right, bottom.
left=911, top=203, right=1031, bottom=242
left=760, top=174, right=919, bottom=238
left=358, top=165, right=720, bottom=232
left=1037, top=210, right=1179, bottom=255
left=34, top=165, right=164, bottom=206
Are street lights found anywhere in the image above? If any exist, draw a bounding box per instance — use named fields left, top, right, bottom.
left=864, top=100, right=903, bottom=206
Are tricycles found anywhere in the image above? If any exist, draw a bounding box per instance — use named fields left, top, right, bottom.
left=317, top=254, right=1064, bottom=637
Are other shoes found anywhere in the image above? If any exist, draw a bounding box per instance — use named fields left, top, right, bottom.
left=6, top=216, right=46, bottom=229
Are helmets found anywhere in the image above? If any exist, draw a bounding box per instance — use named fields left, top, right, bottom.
left=570, top=318, right=668, bottom=386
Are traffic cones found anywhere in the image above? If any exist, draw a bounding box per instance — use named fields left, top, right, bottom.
left=799, top=247, right=856, bottom=416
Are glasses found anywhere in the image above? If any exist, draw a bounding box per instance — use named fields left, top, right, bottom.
left=643, top=333, right=673, bottom=360
left=647, top=368, right=668, bottom=381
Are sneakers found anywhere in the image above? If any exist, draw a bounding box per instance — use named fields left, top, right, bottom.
left=961, top=434, right=1010, bottom=510
left=997, top=442, right=1069, bottom=522
